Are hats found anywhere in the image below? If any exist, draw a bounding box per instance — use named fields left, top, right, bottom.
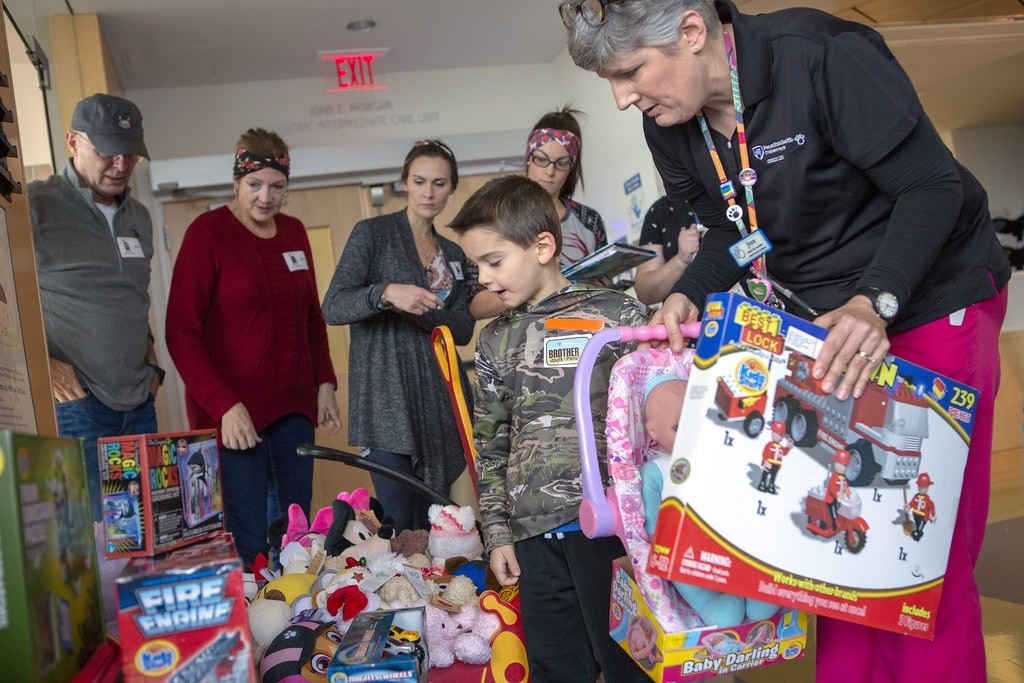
left=72, top=92, right=150, bottom=161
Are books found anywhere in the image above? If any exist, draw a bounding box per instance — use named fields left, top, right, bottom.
left=561, top=241, right=658, bottom=288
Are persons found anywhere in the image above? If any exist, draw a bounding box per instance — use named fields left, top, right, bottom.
left=26, top=93, right=165, bottom=517
left=167, top=128, right=342, bottom=565
left=524, top=101, right=614, bottom=291
left=323, top=139, right=476, bottom=533
left=642, top=384, right=782, bottom=628
left=446, top=180, right=663, bottom=682
left=558, top=0, right=1009, bottom=683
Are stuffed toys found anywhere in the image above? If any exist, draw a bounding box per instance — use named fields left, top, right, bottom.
left=236, top=488, right=506, bottom=683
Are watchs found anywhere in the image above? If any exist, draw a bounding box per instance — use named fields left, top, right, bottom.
left=853, top=287, right=898, bottom=326
left=147, top=362, right=166, bottom=384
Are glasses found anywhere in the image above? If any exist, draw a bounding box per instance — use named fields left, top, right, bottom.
left=558, top=0, right=624, bottom=27
left=531, top=152, right=575, bottom=172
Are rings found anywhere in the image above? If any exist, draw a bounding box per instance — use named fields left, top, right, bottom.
left=856, top=350, right=874, bottom=362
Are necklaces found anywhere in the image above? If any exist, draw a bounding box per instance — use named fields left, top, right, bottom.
left=410, top=229, right=435, bottom=264
